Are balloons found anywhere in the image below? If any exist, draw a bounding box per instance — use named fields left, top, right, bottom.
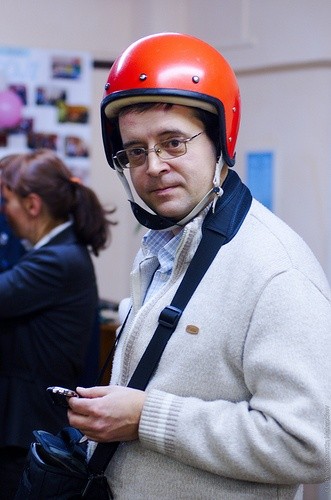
left=0, top=91, right=23, bottom=128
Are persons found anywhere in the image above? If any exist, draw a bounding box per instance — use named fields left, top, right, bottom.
left=65, top=31, right=331, bottom=499
left=1, top=150, right=118, bottom=499
left=0, top=153, right=36, bottom=276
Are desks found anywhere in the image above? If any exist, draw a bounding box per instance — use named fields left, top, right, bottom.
left=98, top=324, right=120, bottom=386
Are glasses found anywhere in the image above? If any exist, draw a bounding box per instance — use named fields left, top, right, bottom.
left=115, top=128, right=217, bottom=169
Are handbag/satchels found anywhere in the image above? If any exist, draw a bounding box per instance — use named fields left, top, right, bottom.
left=12, top=428, right=88, bottom=500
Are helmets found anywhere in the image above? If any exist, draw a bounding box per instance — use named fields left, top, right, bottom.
left=98, top=33, right=240, bottom=168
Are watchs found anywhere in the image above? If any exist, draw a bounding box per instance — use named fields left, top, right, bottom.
left=45, top=386, right=81, bottom=422
